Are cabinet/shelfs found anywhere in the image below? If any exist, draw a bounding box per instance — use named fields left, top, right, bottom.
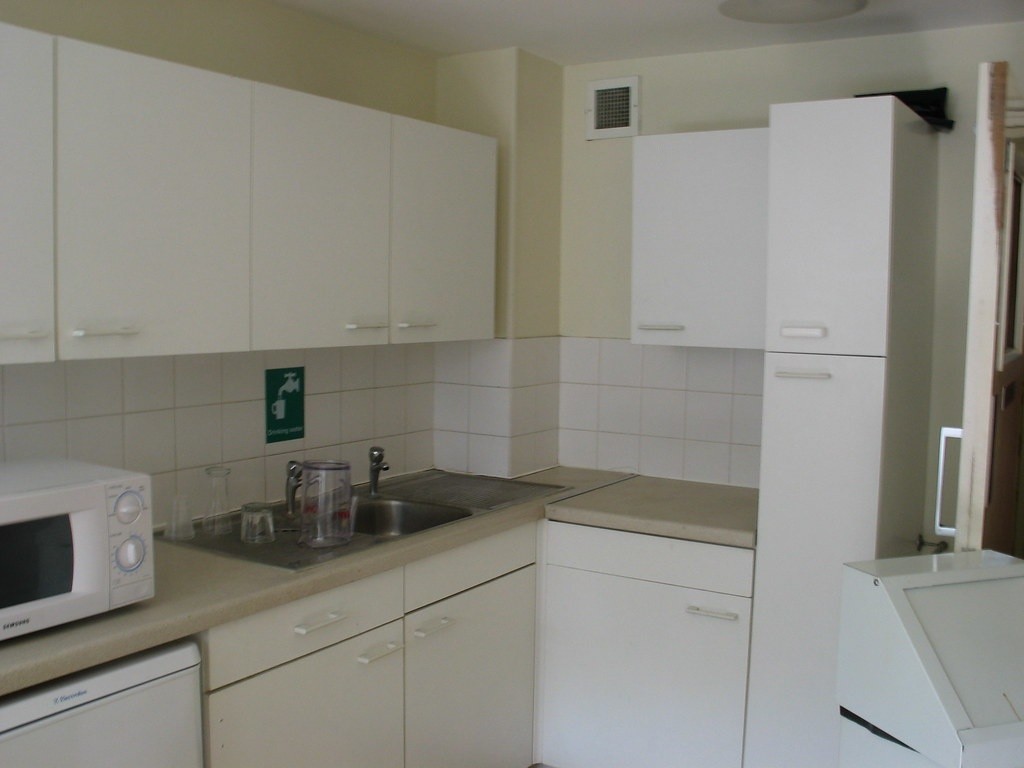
left=743, top=96, right=933, bottom=768
left=200, top=520, right=542, bottom=768
left=536, top=522, right=756, bottom=768
left=629, top=97, right=768, bottom=356
left=0, top=22, right=498, bottom=365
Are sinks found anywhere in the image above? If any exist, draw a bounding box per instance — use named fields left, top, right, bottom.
left=309, top=500, right=473, bottom=537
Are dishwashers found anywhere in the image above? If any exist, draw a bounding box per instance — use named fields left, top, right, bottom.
left=0, top=643, right=204, bottom=768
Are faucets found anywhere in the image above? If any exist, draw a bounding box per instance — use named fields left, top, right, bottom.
left=370, top=445, right=390, bottom=497
left=285, top=458, right=305, bottom=519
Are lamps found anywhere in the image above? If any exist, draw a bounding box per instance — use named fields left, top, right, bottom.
left=719, top=0, right=866, bottom=24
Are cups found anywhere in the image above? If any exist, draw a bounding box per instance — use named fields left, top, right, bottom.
left=202, top=467, right=234, bottom=535
left=239, top=502, right=276, bottom=544
left=271, top=400, right=285, bottom=419
left=300, top=459, right=353, bottom=549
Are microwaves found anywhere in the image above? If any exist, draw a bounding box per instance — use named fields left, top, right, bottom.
left=0, top=457, right=156, bottom=640
